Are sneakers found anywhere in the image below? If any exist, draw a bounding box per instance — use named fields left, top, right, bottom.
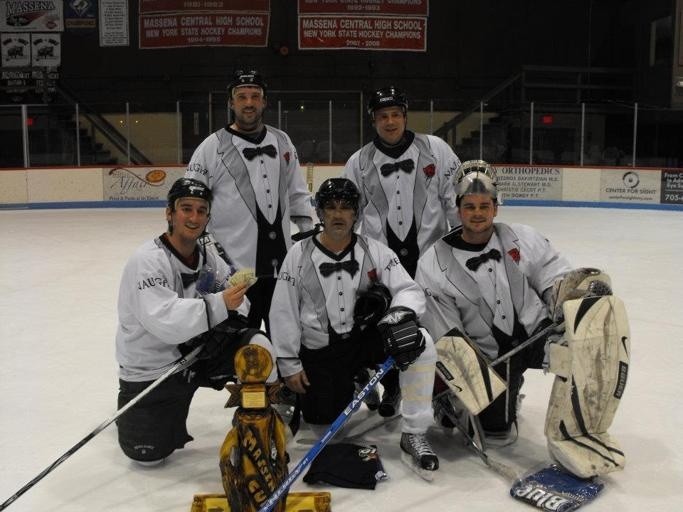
left=378, top=387, right=402, bottom=416
left=432, top=396, right=457, bottom=428
left=400, top=433, right=439, bottom=470
left=363, top=387, right=380, bottom=412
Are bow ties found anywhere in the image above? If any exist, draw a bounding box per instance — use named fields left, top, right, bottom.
left=465, top=249, right=502, bottom=272
left=243, top=144, right=277, bottom=160
left=319, top=260, right=359, bottom=279
left=180, top=269, right=200, bottom=289
left=380, top=159, right=414, bottom=176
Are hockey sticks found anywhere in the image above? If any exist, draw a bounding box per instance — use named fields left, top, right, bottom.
left=297, top=318, right=564, bottom=445
left=435, top=399, right=517, bottom=480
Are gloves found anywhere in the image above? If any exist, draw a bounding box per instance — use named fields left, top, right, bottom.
left=376, top=306, right=426, bottom=370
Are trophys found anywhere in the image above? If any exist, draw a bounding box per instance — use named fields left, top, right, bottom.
left=191, top=342, right=333, bottom=512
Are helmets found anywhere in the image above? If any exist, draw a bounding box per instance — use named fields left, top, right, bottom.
left=368, top=88, right=408, bottom=118
left=316, top=178, right=360, bottom=210
left=452, top=160, right=498, bottom=206
left=228, top=71, right=268, bottom=101
left=168, top=177, right=212, bottom=215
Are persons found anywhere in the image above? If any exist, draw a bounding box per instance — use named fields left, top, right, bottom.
left=267, top=175, right=441, bottom=472
left=114, top=175, right=279, bottom=467
left=340, top=86, right=464, bottom=430
left=182, top=65, right=315, bottom=342
left=414, top=158, right=629, bottom=479
